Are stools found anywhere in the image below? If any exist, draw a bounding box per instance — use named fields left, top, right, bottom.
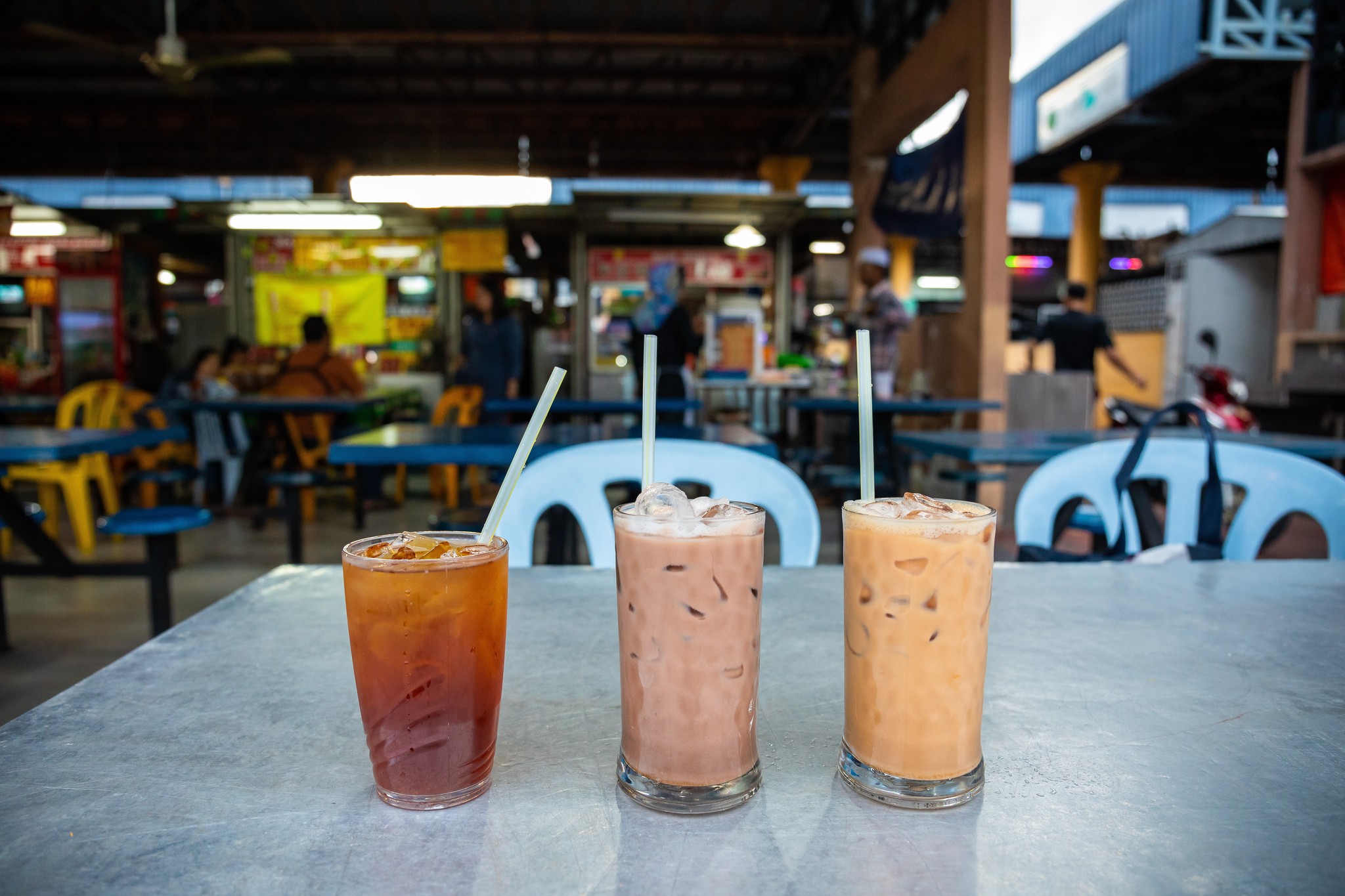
left=97, top=510, right=212, bottom=638
left=120, top=466, right=202, bottom=566
left=938, top=464, right=1007, bottom=504
left=258, top=471, right=322, bottom=564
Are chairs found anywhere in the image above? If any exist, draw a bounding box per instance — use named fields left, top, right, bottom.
left=0, top=382, right=1345, bottom=568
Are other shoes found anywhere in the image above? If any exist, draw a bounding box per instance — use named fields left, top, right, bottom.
left=361, top=495, right=400, bottom=511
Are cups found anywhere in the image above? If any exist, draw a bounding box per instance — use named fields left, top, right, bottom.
left=837, top=498, right=998, bottom=809
left=341, top=531, right=509, bottom=811
left=612, top=501, right=766, bottom=814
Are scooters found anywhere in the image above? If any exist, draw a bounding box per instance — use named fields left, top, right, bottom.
left=1103, top=327, right=1261, bottom=437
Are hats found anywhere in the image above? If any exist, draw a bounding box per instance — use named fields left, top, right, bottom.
left=1227, top=380, right=1250, bottom=405
left=856, top=247, right=891, bottom=268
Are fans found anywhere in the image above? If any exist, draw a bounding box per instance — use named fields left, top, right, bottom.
left=25, top=0, right=295, bottom=86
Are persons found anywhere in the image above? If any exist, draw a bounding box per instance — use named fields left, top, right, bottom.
left=1025, top=283, right=1147, bottom=399
left=274, top=312, right=397, bottom=511
left=830, top=248, right=910, bottom=390
left=447, top=273, right=522, bottom=398
left=176, top=334, right=253, bottom=402
left=627, top=263, right=705, bottom=431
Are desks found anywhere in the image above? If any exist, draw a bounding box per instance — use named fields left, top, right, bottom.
left=0, top=563, right=1345, bottom=896
left=331, top=426, right=779, bottom=547
left=481, top=392, right=703, bottom=422
left=788, top=396, right=1000, bottom=496
left=892, top=425, right=1345, bottom=494
left=0, top=429, right=187, bottom=576
